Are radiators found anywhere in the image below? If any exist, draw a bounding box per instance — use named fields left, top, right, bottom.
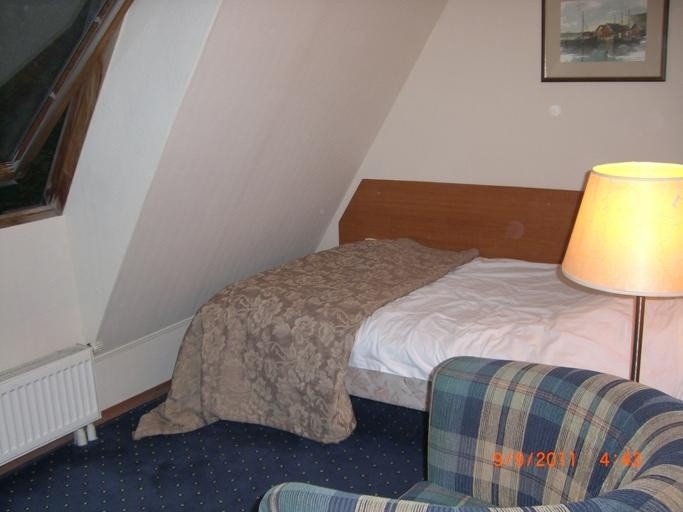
left=0, top=344, right=103, bottom=469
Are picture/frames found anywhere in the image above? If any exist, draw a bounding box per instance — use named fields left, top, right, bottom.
left=540, top=1, right=668, bottom=82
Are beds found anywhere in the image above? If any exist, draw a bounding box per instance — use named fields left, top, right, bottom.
left=193, top=238, right=682, bottom=414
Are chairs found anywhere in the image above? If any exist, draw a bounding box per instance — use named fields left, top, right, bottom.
left=255, top=353, right=682, bottom=512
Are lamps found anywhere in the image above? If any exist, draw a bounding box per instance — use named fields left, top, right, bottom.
left=554, top=162, right=683, bottom=384
